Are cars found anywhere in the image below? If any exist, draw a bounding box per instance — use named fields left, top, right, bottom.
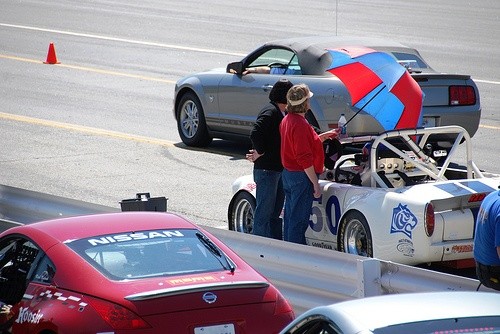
left=280, top=291, right=500, bottom=334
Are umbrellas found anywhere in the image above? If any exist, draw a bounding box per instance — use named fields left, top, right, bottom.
left=324, top=46, right=425, bottom=147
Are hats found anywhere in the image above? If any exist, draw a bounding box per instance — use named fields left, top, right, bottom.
left=286, top=84, right=314, bottom=106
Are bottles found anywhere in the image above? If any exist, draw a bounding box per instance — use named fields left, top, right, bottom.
left=338, top=114, right=347, bottom=140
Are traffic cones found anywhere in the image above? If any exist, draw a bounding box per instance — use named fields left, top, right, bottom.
left=42, top=42, right=61, bottom=64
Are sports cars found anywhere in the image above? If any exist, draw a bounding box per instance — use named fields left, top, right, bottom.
left=0, top=210, right=297, bottom=334
left=171, top=37, right=483, bottom=147
left=227, top=125, right=500, bottom=266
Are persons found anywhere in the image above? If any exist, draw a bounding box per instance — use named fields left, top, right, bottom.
left=242, top=66, right=302, bottom=75
left=473, top=183, right=500, bottom=291
left=246, top=79, right=293, bottom=240
left=280, top=84, right=340, bottom=243
